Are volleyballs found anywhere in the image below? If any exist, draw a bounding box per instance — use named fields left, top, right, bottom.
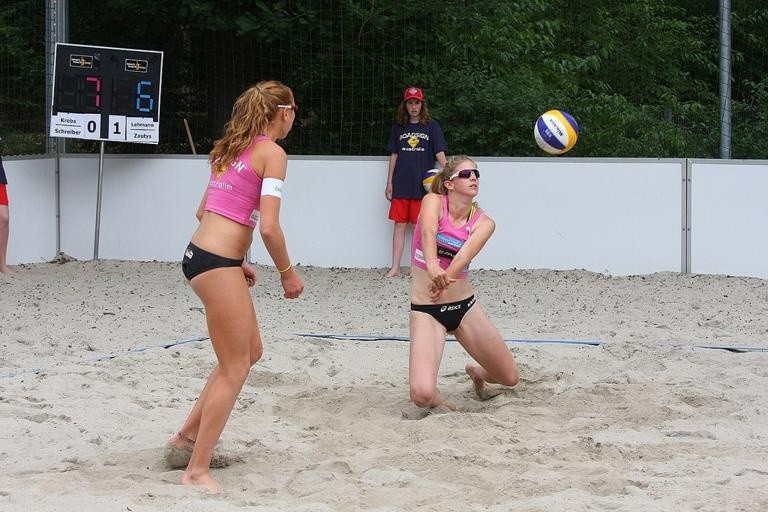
left=534, top=110, right=579, bottom=153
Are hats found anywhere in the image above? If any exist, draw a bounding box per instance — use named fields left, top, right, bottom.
left=404, top=87, right=423, bottom=101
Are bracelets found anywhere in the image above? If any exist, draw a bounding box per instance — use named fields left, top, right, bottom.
left=280, top=264, right=292, bottom=272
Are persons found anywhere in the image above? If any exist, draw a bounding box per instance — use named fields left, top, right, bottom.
left=409, top=155, right=519, bottom=410
left=385, top=88, right=448, bottom=278
left=0, top=156, right=14, bottom=274
left=164, top=81, right=304, bottom=490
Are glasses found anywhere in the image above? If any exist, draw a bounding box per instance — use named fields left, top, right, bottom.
left=278, top=105, right=298, bottom=112
left=450, top=168, right=480, bottom=181
